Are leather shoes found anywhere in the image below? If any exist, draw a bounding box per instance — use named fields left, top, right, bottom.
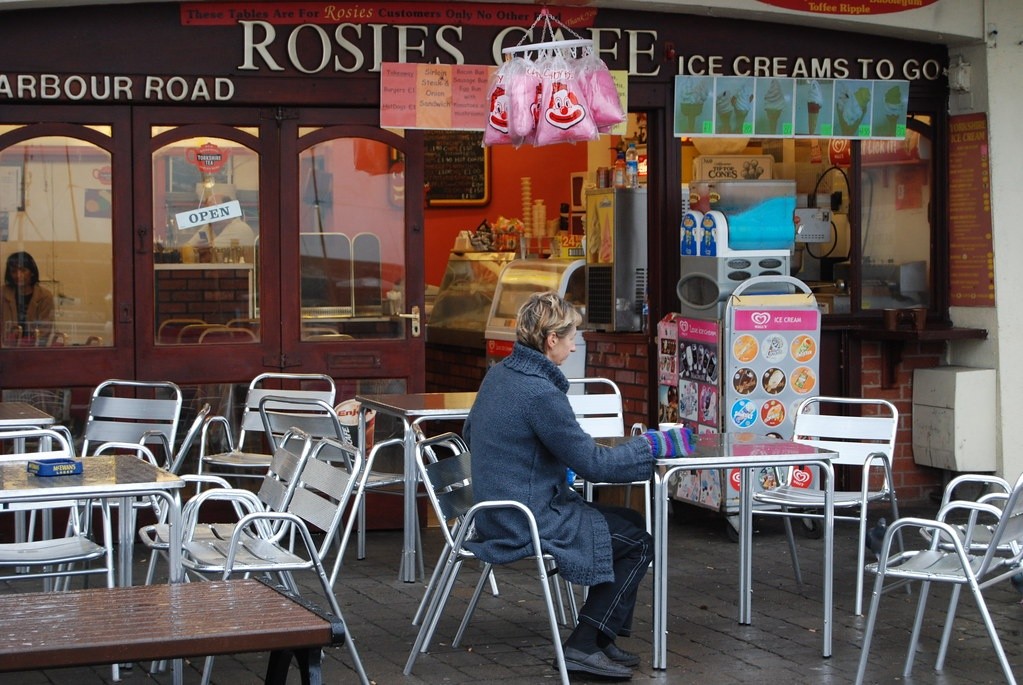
left=598, top=641, right=641, bottom=666
left=553, top=644, right=633, bottom=678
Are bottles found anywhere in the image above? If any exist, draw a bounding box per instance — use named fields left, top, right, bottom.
left=613, top=143, right=639, bottom=191
left=193, top=231, right=213, bottom=263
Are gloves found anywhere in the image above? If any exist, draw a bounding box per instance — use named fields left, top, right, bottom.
left=567, top=468, right=577, bottom=486
left=642, top=428, right=697, bottom=458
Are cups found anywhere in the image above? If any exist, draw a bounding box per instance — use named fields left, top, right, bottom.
left=193, top=243, right=256, bottom=263
left=658, top=422, right=684, bottom=431
left=334, top=398, right=377, bottom=473
left=882, top=307, right=928, bottom=331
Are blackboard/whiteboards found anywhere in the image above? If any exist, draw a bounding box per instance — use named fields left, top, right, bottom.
left=419, top=129, right=491, bottom=206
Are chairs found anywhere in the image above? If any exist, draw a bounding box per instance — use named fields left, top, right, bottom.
left=228, top=317, right=357, bottom=342
left=401, top=430, right=586, bottom=685
left=47, top=331, right=70, bottom=347
left=6, top=325, right=23, bottom=346
left=855, top=469, right=1023, bottom=685
left=200, top=329, right=254, bottom=344
left=560, top=377, right=655, bottom=570
left=746, top=396, right=915, bottom=618
left=0, top=373, right=364, bottom=685
left=176, top=324, right=233, bottom=342
left=158, top=319, right=206, bottom=343
left=85, top=336, right=102, bottom=345
left=919, top=471, right=1022, bottom=563
left=27, top=329, right=42, bottom=346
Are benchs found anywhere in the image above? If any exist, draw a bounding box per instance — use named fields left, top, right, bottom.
left=0, top=575, right=347, bottom=685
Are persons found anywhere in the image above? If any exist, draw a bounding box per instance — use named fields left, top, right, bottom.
left=0, top=251, right=55, bottom=348
left=462, top=292, right=695, bottom=677
left=186, top=193, right=255, bottom=264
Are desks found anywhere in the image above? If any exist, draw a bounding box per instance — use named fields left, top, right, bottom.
left=0, top=400, right=55, bottom=451
left=580, top=433, right=839, bottom=671
left=356, top=391, right=477, bottom=583
left=0, top=453, right=181, bottom=593
